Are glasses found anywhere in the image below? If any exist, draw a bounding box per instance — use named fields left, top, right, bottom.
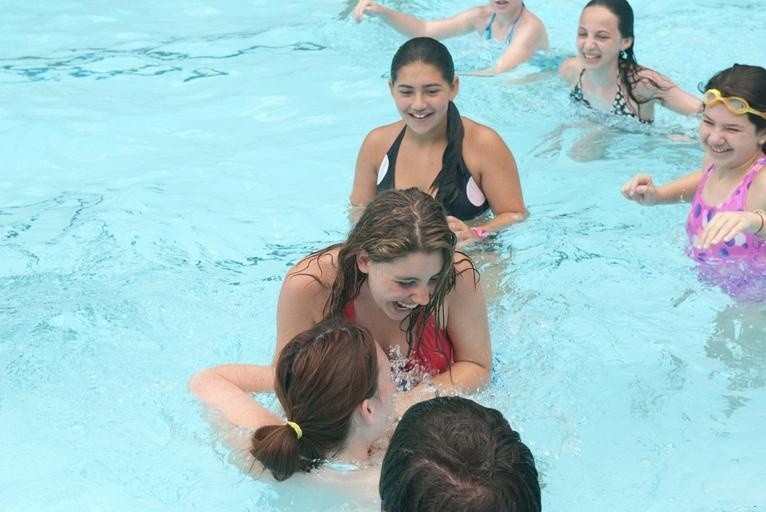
left=701, top=89, right=766, bottom=120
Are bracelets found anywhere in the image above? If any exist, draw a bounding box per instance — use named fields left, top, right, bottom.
left=471, top=225, right=487, bottom=241
left=425, top=377, right=443, bottom=400
left=755, top=210, right=764, bottom=238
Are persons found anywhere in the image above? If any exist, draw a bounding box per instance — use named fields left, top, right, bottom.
left=345, top=34, right=529, bottom=305
left=619, top=64, right=765, bottom=305
left=354, top=0, right=552, bottom=80
left=186, top=320, right=394, bottom=498
left=505, top=0, right=707, bottom=171
left=278, top=186, right=494, bottom=437
left=375, top=394, right=543, bottom=512
